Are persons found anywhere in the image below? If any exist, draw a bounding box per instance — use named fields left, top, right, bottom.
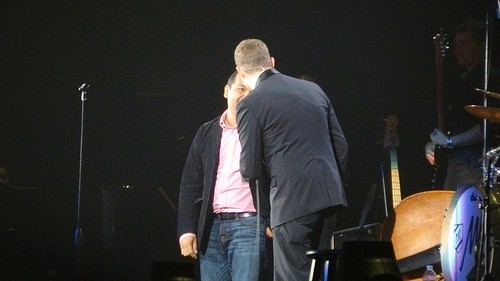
left=235, top=38, right=350, bottom=281
left=425, top=20, right=500, bottom=281
left=178, top=70, right=272, bottom=281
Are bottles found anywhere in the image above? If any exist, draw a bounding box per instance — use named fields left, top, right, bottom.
left=423, top=266, right=437, bottom=281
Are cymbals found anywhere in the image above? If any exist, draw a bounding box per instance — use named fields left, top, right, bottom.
left=471, top=85, right=500, bottom=98
left=465, top=104, right=500, bottom=125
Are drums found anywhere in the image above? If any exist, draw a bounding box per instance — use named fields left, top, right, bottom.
left=437, top=177, right=500, bottom=280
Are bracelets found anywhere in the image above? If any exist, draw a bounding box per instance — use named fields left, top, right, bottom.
left=447, top=136, right=453, bottom=149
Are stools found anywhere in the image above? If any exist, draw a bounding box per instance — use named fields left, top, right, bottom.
left=305, top=250, right=341, bottom=281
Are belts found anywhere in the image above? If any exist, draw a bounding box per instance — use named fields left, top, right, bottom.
left=209, top=212, right=256, bottom=219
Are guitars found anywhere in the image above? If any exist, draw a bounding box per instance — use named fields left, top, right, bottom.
left=428, top=29, right=452, bottom=166
left=381, top=113, right=403, bottom=207
